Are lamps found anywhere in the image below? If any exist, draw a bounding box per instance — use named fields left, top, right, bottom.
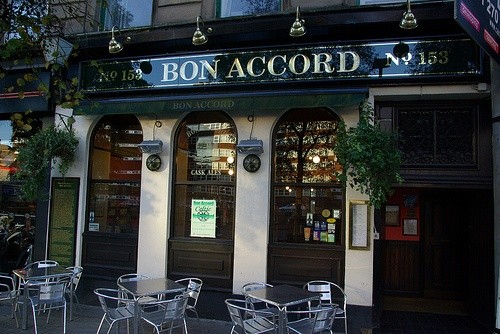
left=107, top=25, right=124, bottom=53
left=399, top=0, right=419, bottom=29
left=289, top=6, right=307, bottom=37
left=137, top=140, right=163, bottom=154
left=192, top=15, right=212, bottom=45
left=236, top=140, right=264, bottom=154
left=243, top=155, right=264, bottom=172
left=146, top=154, right=161, bottom=171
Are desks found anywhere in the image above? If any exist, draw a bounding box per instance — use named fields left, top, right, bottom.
left=244, top=284, right=322, bottom=334
left=11, top=267, right=75, bottom=329
left=117, top=278, right=187, bottom=334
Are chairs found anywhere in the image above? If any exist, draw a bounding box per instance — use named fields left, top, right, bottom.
left=0, top=260, right=348, bottom=334
